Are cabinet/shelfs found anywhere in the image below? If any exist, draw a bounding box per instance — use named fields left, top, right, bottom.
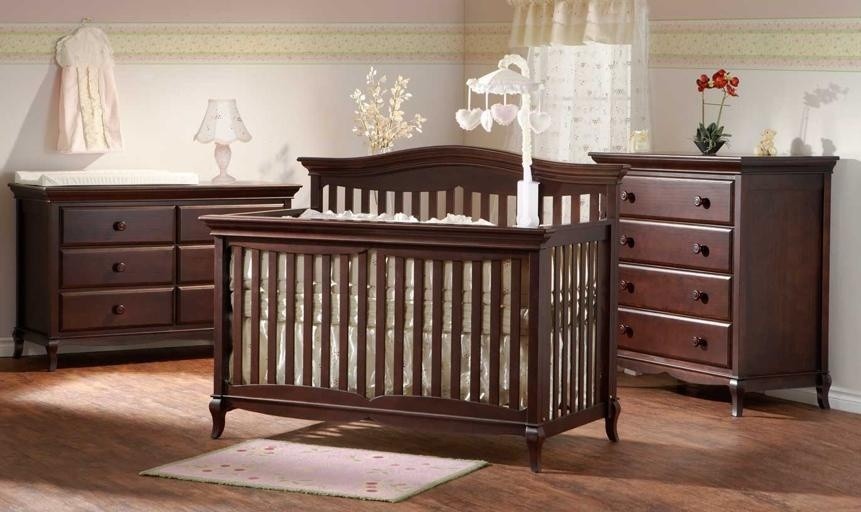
left=588, top=151, right=840, bottom=418
left=6, top=183, right=303, bottom=373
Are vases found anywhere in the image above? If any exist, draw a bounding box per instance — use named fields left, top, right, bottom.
left=694, top=141, right=726, bottom=156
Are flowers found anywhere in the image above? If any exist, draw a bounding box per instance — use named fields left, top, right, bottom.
left=688, top=69, right=740, bottom=152
left=348, top=65, right=427, bottom=153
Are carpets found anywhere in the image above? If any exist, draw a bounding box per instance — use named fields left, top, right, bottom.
left=138, top=437, right=490, bottom=504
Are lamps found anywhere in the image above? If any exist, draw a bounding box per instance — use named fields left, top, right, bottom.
left=192, top=99, right=252, bottom=182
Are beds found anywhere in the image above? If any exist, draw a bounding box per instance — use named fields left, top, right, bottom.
left=196, top=145, right=633, bottom=473
